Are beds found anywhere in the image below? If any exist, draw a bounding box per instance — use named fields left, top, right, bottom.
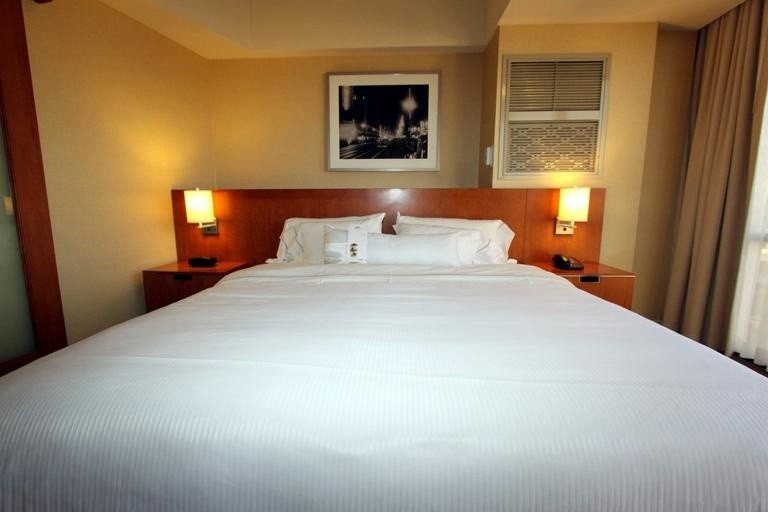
left=0, top=183, right=768, bottom=512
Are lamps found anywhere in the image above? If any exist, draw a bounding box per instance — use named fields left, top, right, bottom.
left=183, top=187, right=218, bottom=234
left=554, top=182, right=590, bottom=235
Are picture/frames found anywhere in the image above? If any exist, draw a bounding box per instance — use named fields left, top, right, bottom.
left=327, top=69, right=440, bottom=171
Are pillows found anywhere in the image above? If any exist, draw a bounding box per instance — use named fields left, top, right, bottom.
left=263, top=212, right=517, bottom=266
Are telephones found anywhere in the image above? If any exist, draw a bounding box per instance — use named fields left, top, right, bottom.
left=553, top=254, right=584, bottom=270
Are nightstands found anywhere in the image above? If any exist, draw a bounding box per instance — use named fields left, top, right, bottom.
left=525, top=261, right=637, bottom=311
left=143, top=260, right=248, bottom=309
